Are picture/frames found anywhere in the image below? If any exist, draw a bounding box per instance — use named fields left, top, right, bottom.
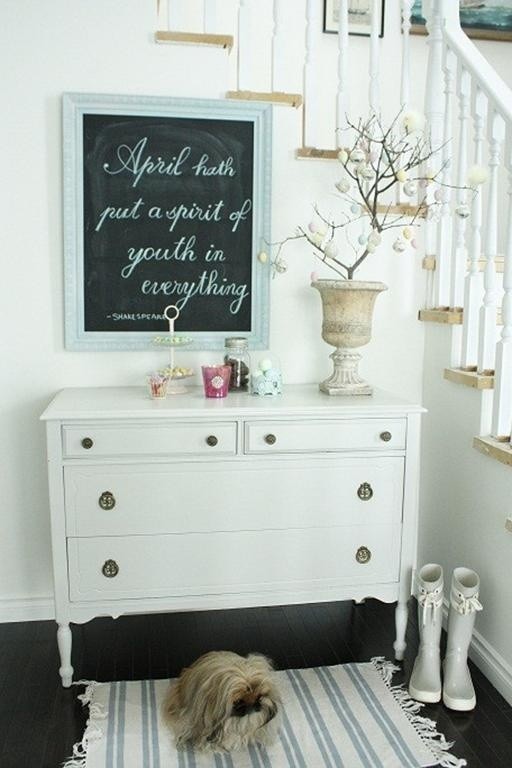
left=321, top=2, right=511, bottom=44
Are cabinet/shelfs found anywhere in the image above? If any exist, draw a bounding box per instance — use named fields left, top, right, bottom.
left=38, top=385, right=428, bottom=690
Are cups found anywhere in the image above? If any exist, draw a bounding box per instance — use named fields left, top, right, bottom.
left=202, top=362, right=231, bottom=398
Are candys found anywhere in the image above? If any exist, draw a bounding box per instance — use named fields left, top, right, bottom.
left=159, top=366, right=195, bottom=378
left=154, top=336, right=192, bottom=345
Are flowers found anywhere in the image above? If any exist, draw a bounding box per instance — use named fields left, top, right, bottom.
left=257, top=98, right=490, bottom=282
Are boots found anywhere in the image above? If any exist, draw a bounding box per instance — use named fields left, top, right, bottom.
left=407, top=564, right=443, bottom=703
left=439, top=567, right=483, bottom=712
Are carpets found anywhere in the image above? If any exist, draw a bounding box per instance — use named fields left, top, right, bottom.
left=63, top=656, right=469, bottom=768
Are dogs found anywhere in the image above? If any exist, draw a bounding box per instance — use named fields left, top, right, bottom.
left=160, top=651, right=284, bottom=754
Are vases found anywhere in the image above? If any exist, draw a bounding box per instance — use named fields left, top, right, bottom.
left=314, top=281, right=388, bottom=397
